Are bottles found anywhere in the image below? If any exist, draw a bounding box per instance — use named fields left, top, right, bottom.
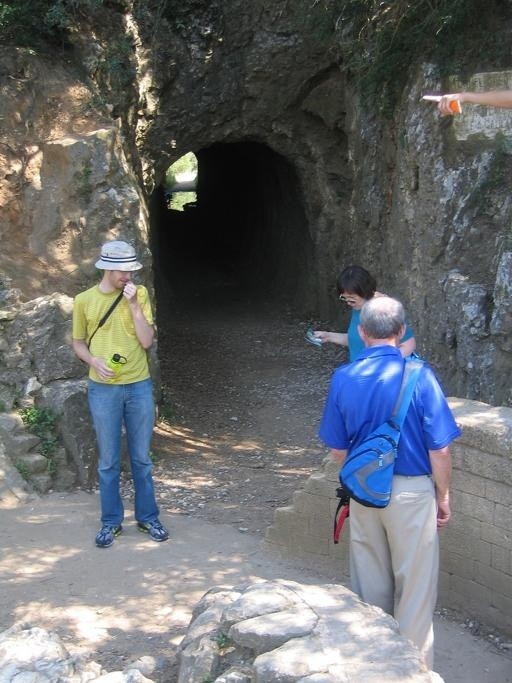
left=104, top=353, right=127, bottom=383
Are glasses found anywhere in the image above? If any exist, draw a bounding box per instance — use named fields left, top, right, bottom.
left=339, top=295, right=357, bottom=302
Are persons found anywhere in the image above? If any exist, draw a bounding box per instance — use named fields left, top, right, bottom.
left=318, top=295, right=464, bottom=670
left=71, top=240, right=170, bottom=547
left=305, top=264, right=417, bottom=364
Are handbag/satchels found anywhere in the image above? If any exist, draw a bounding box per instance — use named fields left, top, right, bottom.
left=339, top=420, right=401, bottom=508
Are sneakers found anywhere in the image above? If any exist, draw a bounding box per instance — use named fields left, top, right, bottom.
left=96, top=525, right=121, bottom=547
left=137, top=518, right=169, bottom=541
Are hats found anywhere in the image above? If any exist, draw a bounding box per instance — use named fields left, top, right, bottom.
left=95, top=241, right=143, bottom=272
